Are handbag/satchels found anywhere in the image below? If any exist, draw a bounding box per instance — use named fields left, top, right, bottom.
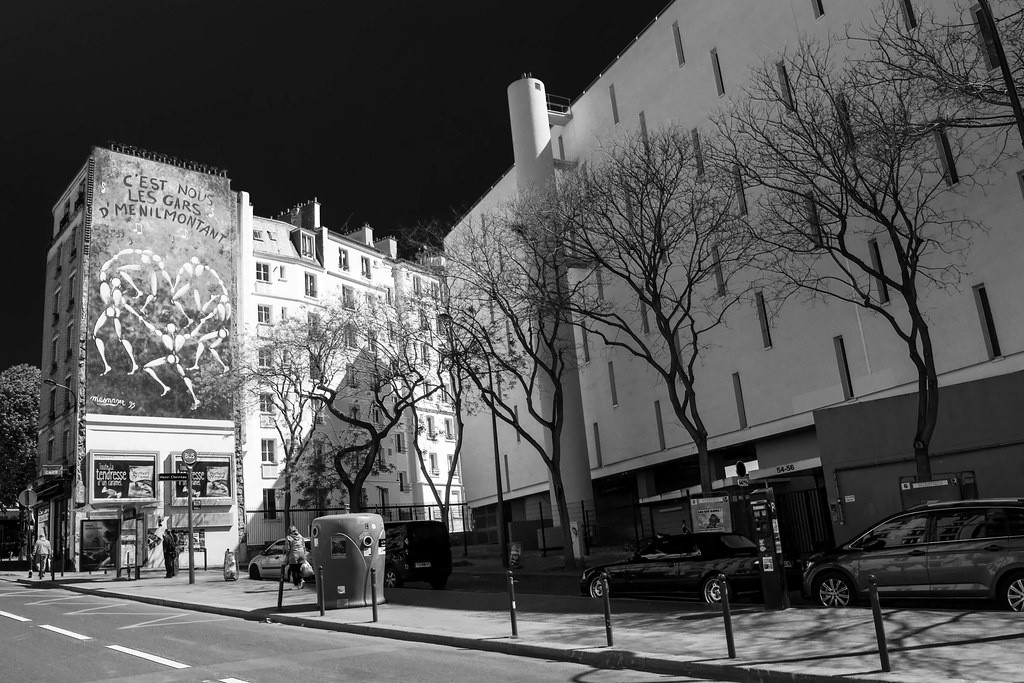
left=48, top=558, right=51, bottom=571
left=301, top=559, right=314, bottom=577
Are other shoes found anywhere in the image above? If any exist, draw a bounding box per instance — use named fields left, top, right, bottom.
left=164, top=576, right=171, bottom=578
left=292, top=587, right=299, bottom=590
left=299, top=581, right=306, bottom=588
left=38, top=574, right=44, bottom=577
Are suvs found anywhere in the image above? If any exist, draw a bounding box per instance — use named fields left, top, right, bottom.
left=383, top=520, right=454, bottom=590
left=800, top=496, right=1024, bottom=612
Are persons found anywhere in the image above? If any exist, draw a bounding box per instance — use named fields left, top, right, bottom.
left=282, top=525, right=306, bottom=586
left=32, top=534, right=52, bottom=577
left=162, top=529, right=180, bottom=578
left=680, top=519, right=691, bottom=534
left=100, top=530, right=116, bottom=567
left=706, top=514, right=720, bottom=528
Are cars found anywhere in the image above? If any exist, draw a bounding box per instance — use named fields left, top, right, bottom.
left=578, top=531, right=784, bottom=611
left=247, top=536, right=316, bottom=581
